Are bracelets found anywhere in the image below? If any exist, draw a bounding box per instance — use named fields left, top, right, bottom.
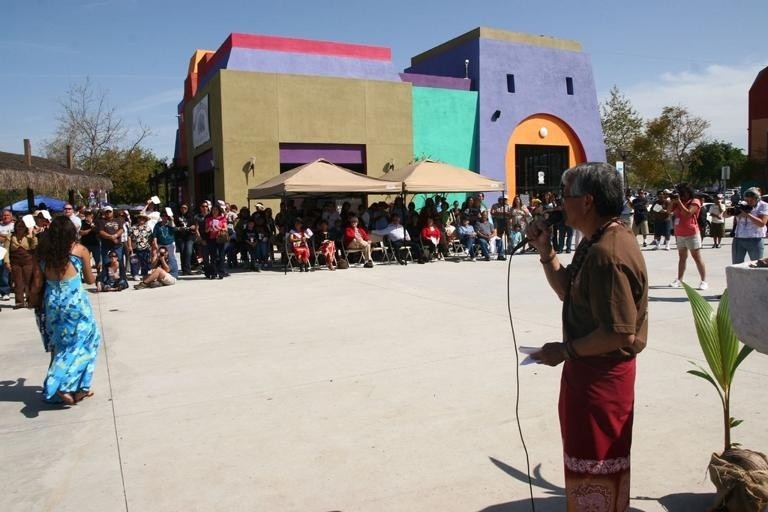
left=565, top=340, right=578, bottom=358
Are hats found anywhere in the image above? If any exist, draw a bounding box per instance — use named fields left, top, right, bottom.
left=84, top=209, right=92, bottom=214
left=663, top=189, right=673, bottom=193
left=103, top=206, right=113, bottom=213
left=134, top=213, right=151, bottom=221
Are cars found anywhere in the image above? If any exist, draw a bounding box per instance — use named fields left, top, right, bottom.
left=694, top=185, right=741, bottom=203
left=701, top=202, right=737, bottom=237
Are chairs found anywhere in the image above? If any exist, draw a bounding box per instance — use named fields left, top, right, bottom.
left=286, top=232, right=482, bottom=272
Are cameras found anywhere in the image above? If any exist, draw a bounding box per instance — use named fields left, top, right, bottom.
left=669, top=190, right=680, bottom=200
left=160, top=252, right=165, bottom=258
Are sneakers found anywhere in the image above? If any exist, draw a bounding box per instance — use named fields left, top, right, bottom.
left=567, top=249, right=571, bottom=252
left=670, top=279, right=684, bottom=287
left=364, top=260, right=372, bottom=267
left=712, top=244, right=720, bottom=248
left=498, top=256, right=506, bottom=260
left=698, top=282, right=707, bottom=289
left=486, top=257, right=490, bottom=261
left=557, top=250, right=562, bottom=253
left=150, top=282, right=163, bottom=288
left=135, top=282, right=150, bottom=289
left=219, top=272, right=228, bottom=279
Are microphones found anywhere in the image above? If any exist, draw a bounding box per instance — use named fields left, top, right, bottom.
left=512, top=210, right=565, bottom=256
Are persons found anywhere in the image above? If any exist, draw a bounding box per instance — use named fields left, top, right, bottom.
left=175, top=188, right=573, bottom=280
left=1, top=197, right=179, bottom=308
left=722, top=187, right=768, bottom=264
left=29, top=233, right=56, bottom=395
left=526, top=163, right=649, bottom=510
left=666, top=185, right=709, bottom=288
left=26, top=215, right=100, bottom=404
left=625, top=189, right=740, bottom=247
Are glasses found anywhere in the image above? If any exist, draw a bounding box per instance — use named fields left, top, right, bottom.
left=561, top=195, right=580, bottom=204
left=64, top=208, right=71, bottom=210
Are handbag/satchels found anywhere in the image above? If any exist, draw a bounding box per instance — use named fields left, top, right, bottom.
left=338, top=259, right=348, bottom=268
left=191, top=231, right=200, bottom=241
left=131, top=235, right=137, bottom=249
left=216, top=230, right=229, bottom=244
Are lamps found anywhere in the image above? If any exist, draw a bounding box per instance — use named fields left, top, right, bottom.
left=250, top=156, right=256, bottom=177
left=389, top=158, right=395, bottom=171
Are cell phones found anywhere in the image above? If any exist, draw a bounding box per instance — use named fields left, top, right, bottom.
left=213, top=220, right=220, bottom=231
